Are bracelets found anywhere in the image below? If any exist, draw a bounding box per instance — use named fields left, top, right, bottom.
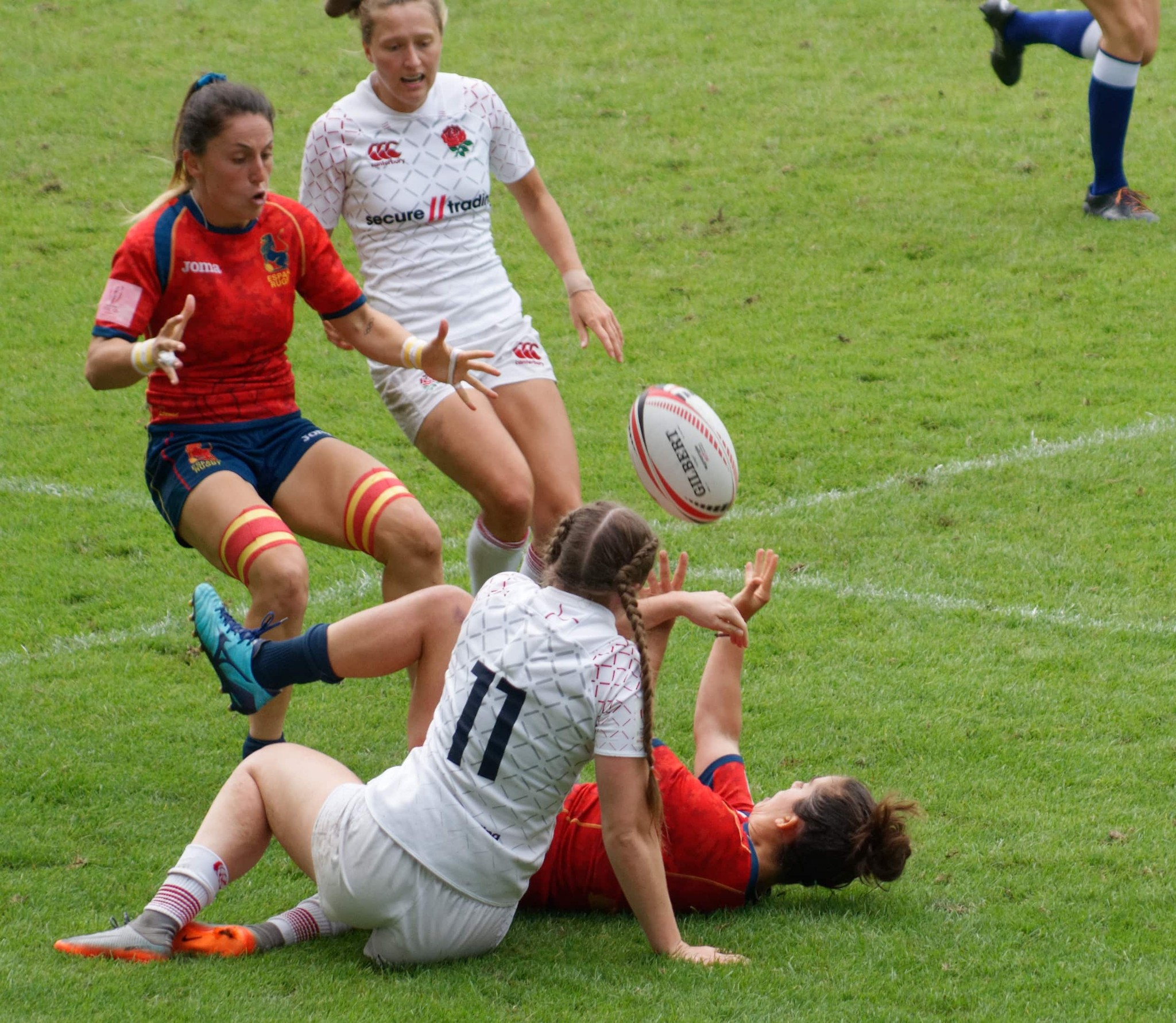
left=563, top=270, right=594, bottom=297
left=714, top=633, right=730, bottom=637
left=131, top=338, right=159, bottom=377
left=400, top=336, right=427, bottom=370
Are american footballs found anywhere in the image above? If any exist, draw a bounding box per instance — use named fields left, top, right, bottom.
left=625, top=382, right=737, bottom=525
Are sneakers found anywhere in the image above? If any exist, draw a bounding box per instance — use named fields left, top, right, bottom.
left=172, top=920, right=285, bottom=957
left=1083, top=183, right=1160, bottom=224
left=54, top=910, right=179, bottom=964
left=188, top=583, right=289, bottom=715
left=979, top=0, right=1025, bottom=86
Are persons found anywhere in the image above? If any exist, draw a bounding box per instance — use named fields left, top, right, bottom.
left=85, top=70, right=502, bottom=760
left=191, top=547, right=917, bottom=915
left=981, top=0, right=1160, bottom=224
left=53, top=502, right=750, bottom=966
left=299, top=0, right=627, bottom=593
